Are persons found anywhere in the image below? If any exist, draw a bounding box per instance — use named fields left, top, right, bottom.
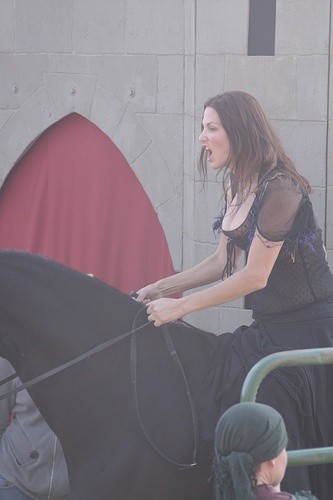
left=130, top=91, right=333, bottom=500
left=213, top=401, right=318, bottom=500
left=0, top=357, right=72, bottom=500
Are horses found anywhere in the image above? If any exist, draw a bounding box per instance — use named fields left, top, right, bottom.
left=0, top=250, right=217, bottom=500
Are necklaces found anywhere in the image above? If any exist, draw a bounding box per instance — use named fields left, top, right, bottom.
left=243, top=186, right=256, bottom=196
